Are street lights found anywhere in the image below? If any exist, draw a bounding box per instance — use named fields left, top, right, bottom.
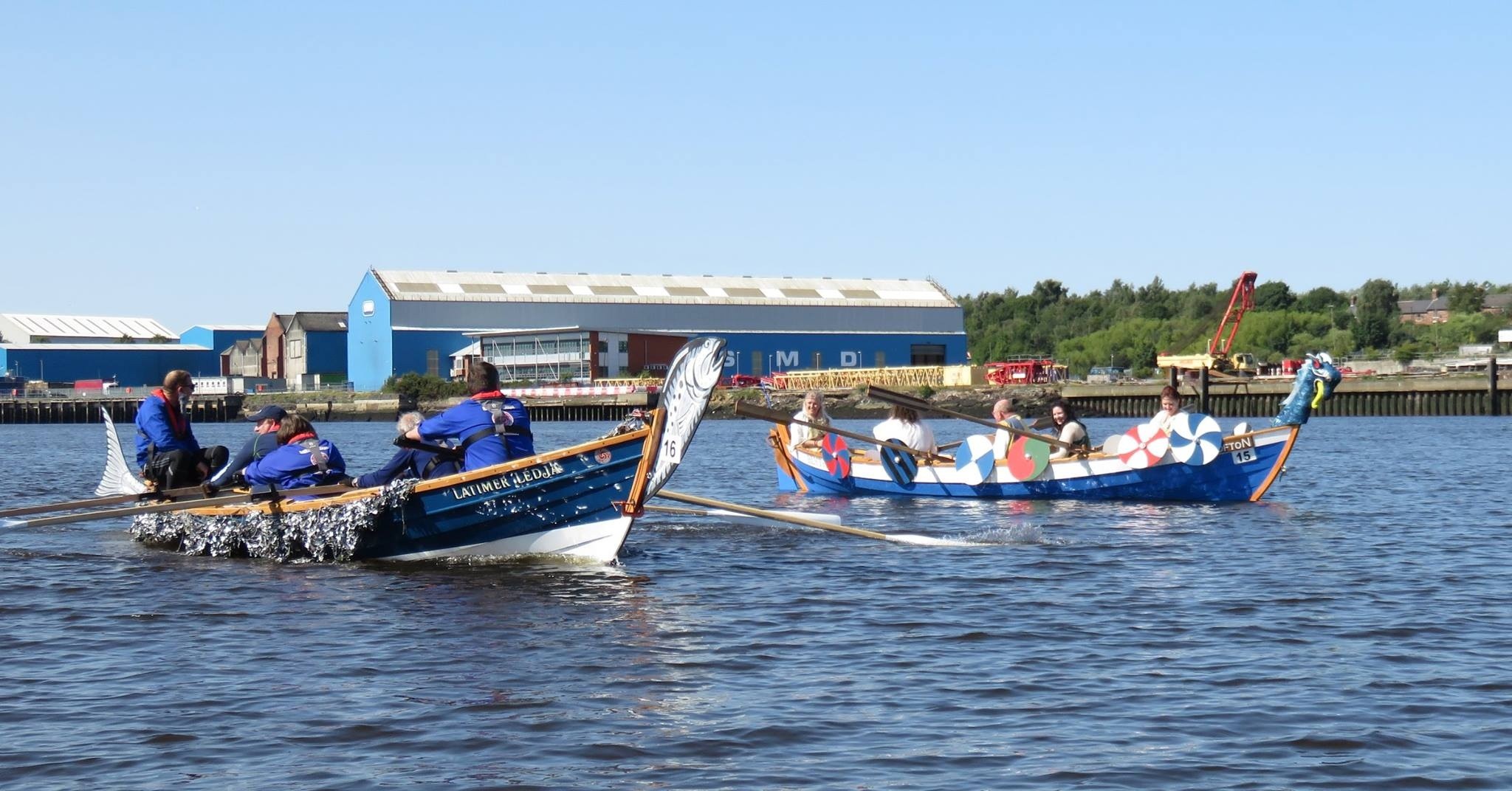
left=1207, top=338, right=1212, bottom=354
left=1221, top=339, right=1226, bottom=352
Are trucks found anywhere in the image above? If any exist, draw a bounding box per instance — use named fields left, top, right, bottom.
left=1157, top=352, right=1256, bottom=374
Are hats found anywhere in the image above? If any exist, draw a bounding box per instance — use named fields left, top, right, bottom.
left=248, top=406, right=287, bottom=422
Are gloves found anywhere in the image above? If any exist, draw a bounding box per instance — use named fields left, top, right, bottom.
left=393, top=432, right=419, bottom=450
left=338, top=476, right=355, bottom=487
left=232, top=470, right=242, bottom=484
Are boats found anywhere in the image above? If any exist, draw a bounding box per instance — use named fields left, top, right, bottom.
left=767, top=347, right=1342, bottom=506
left=89, top=331, right=726, bottom=571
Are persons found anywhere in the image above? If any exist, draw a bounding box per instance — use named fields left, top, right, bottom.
left=1048, top=399, right=1092, bottom=460
left=991, top=399, right=1028, bottom=460
left=1149, top=387, right=1189, bottom=433
left=873, top=391, right=938, bottom=459
left=200, top=406, right=288, bottom=491
left=233, top=414, right=345, bottom=502
left=405, top=362, right=536, bottom=471
left=136, top=371, right=228, bottom=503
left=791, top=390, right=833, bottom=453
left=336, top=411, right=463, bottom=487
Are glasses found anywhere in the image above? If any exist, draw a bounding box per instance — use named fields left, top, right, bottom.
left=180, top=384, right=195, bottom=390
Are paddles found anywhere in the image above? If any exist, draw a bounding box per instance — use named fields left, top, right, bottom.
left=653, top=490, right=954, bottom=544
left=936, top=415, right=1054, bottom=451
left=0, top=483, right=360, bottom=535
left=734, top=400, right=957, bottom=462
left=0, top=482, right=249, bottom=518
left=866, top=384, right=1098, bottom=454
left=643, top=505, right=842, bottom=530
